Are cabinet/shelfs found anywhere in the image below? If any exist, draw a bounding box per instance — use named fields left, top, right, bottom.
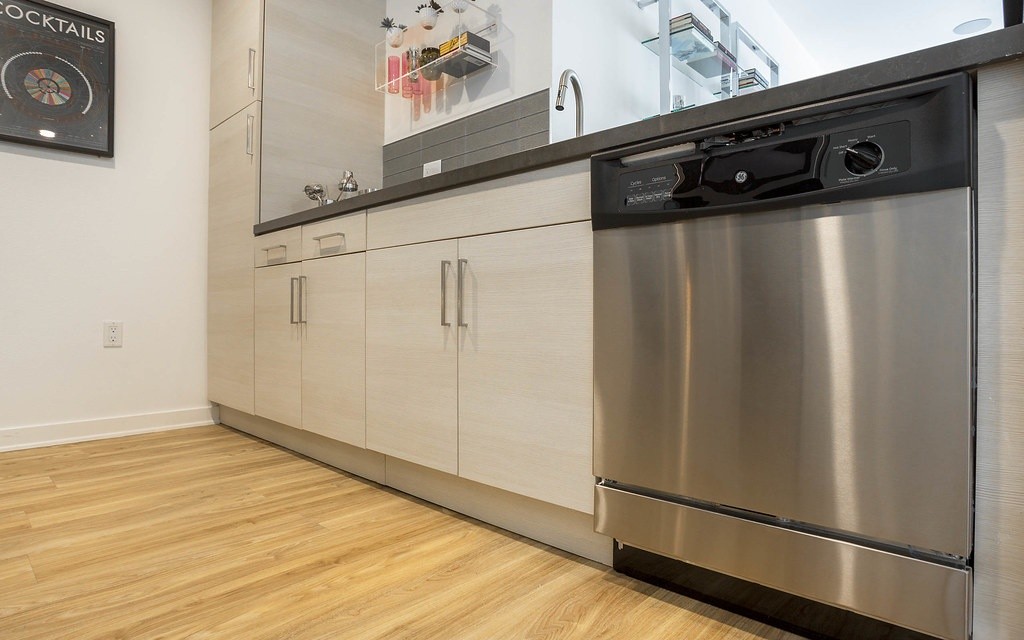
left=374, top=0, right=498, bottom=99
left=255, top=154, right=615, bottom=568
left=638, top=0, right=781, bottom=120
left=210, top=0, right=383, bottom=437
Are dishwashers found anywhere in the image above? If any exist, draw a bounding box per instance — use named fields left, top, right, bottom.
left=587, top=72, right=979, bottom=640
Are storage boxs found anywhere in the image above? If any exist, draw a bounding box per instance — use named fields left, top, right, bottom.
left=434, top=31, right=490, bottom=79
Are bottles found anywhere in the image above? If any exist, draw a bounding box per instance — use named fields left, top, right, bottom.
left=418, top=46, right=442, bottom=81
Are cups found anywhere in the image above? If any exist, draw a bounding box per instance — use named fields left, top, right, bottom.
left=672, top=95, right=685, bottom=109
left=388, top=55, right=400, bottom=93
left=401, top=47, right=423, bottom=98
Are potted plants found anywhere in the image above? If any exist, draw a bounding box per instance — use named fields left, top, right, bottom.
left=415, top=0, right=445, bottom=30
left=387, top=47, right=442, bottom=98
left=380, top=17, right=408, bottom=49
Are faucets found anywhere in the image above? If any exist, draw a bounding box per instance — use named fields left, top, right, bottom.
left=555, top=67, right=585, bottom=142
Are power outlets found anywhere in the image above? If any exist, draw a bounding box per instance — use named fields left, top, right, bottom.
left=103, top=321, right=123, bottom=347
